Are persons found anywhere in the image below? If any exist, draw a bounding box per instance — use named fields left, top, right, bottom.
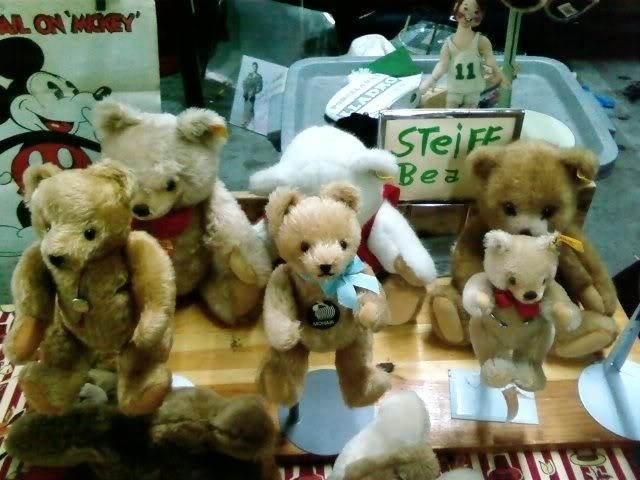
left=239, top=62, right=264, bottom=128
left=416, top=0, right=513, bottom=109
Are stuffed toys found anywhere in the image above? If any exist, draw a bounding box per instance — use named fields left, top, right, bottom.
left=4, top=387, right=282, bottom=480
left=255, top=182, right=390, bottom=405
left=248, top=127, right=437, bottom=325
left=428, top=140, right=620, bottom=360
left=6, top=160, right=177, bottom=415
left=328, top=389, right=441, bottom=479
left=92, top=100, right=270, bottom=323
left=463, top=230, right=583, bottom=391
left=0, top=92, right=622, bottom=480
